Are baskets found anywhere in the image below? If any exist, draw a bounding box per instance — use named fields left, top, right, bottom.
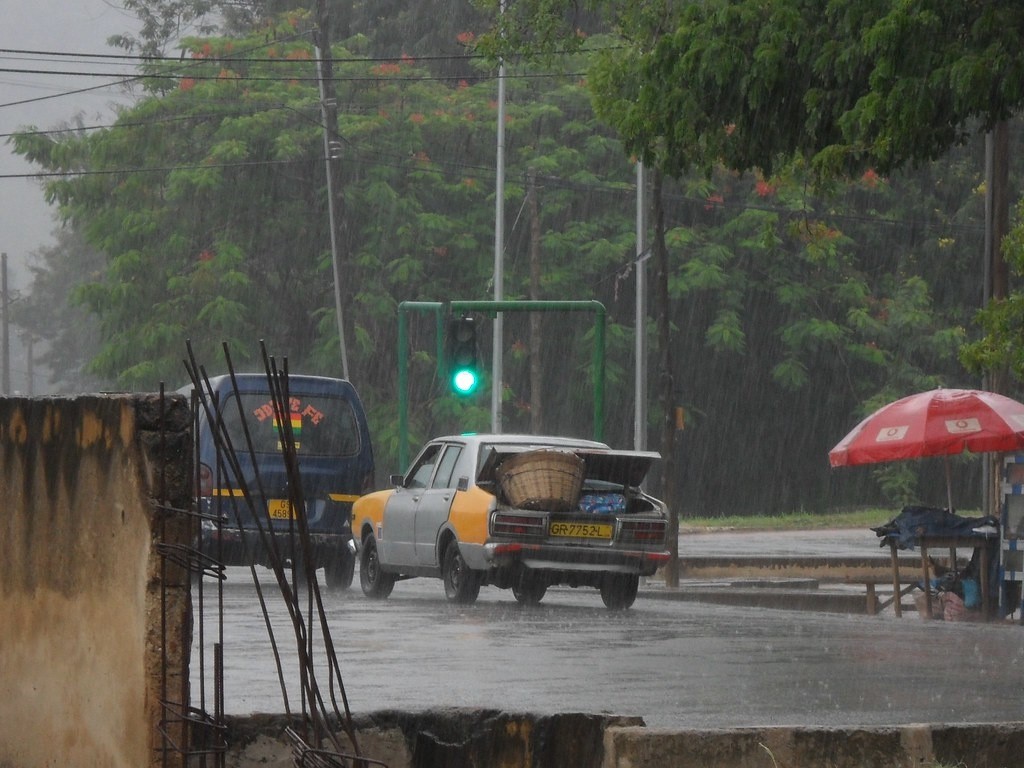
left=494, top=449, right=586, bottom=512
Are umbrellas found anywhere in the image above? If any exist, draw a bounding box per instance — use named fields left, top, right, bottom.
left=827, top=388, right=1023, bottom=587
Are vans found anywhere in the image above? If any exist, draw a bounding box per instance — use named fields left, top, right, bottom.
left=172, top=373, right=376, bottom=590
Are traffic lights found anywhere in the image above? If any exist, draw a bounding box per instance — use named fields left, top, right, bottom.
left=448, top=317, right=480, bottom=398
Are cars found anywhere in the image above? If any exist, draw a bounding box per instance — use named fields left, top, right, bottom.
left=349, top=432, right=673, bottom=610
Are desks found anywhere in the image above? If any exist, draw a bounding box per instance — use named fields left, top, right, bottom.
left=885, top=531, right=999, bottom=622
